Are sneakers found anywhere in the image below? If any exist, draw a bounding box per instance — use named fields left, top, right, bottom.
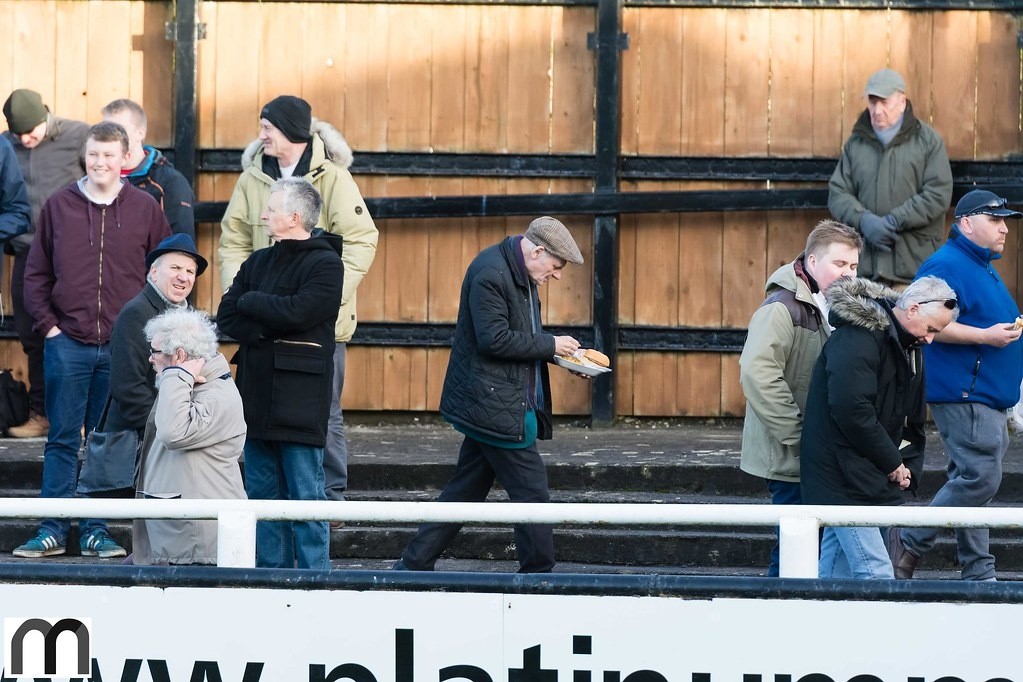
left=12, top=531, right=66, bottom=558
left=80, top=528, right=128, bottom=558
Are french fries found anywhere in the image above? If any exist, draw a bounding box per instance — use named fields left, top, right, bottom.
left=562, top=356, right=580, bottom=363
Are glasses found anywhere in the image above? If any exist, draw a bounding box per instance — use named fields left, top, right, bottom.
left=150, top=349, right=162, bottom=359
left=918, top=297, right=957, bottom=310
left=963, top=198, right=1008, bottom=214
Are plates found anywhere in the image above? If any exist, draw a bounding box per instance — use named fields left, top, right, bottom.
left=553, top=352, right=612, bottom=377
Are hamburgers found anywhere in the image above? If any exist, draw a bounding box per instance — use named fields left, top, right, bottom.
left=582, top=348, right=609, bottom=368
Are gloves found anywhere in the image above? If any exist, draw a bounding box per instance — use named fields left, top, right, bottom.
left=859, top=210, right=899, bottom=253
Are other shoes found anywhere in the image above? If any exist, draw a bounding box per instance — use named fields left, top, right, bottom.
left=9, top=415, right=51, bottom=437
left=330, top=520, right=347, bottom=529
left=392, top=561, right=409, bottom=571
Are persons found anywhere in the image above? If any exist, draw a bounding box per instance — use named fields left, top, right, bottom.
left=884, top=190, right=1023, bottom=581
left=0, top=88, right=380, bottom=570
left=740, top=221, right=864, bottom=577
left=828, top=69, right=951, bottom=425
left=390, top=216, right=584, bottom=572
left=801, top=275, right=959, bottom=579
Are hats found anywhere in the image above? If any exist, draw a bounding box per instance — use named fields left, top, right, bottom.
left=865, top=69, right=906, bottom=99
left=525, top=216, right=585, bottom=267
left=953, top=189, right=1023, bottom=218
left=260, top=95, right=312, bottom=143
left=145, top=233, right=209, bottom=277
left=3, top=89, right=47, bottom=133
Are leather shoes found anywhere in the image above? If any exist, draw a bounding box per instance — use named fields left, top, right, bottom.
left=884, top=525, right=918, bottom=580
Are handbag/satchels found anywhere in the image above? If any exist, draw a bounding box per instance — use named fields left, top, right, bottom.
left=76, top=430, right=143, bottom=498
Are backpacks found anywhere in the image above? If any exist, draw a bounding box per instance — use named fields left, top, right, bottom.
left=0, top=369, right=30, bottom=438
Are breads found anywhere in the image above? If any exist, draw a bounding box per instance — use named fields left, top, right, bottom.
left=1014, top=318, right=1023, bottom=331
